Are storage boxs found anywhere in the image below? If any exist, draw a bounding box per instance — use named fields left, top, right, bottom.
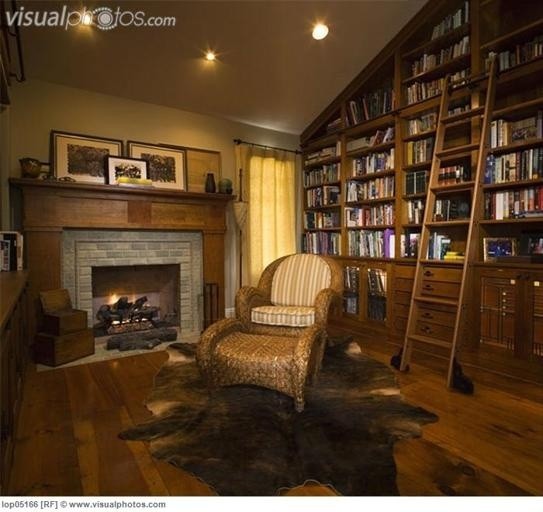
left=32, top=327, right=95, bottom=366
left=39, top=288, right=88, bottom=336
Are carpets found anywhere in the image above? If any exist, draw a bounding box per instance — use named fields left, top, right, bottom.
left=118, top=332, right=439, bottom=496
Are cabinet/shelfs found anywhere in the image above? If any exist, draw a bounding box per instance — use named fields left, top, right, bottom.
left=0, top=271, right=29, bottom=496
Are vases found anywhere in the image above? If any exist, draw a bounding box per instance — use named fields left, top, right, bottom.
left=205, top=172, right=216, bottom=193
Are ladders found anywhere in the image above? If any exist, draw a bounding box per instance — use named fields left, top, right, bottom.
left=397, top=59, right=497, bottom=391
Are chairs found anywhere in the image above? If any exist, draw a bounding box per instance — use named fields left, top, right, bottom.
left=196, top=253, right=348, bottom=413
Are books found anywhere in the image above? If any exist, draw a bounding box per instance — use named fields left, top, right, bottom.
left=0, top=231, right=21, bottom=271
left=339, top=264, right=388, bottom=324
left=114, top=177, right=153, bottom=185
left=118, top=182, right=154, bottom=190
left=300, top=1, right=542, bottom=261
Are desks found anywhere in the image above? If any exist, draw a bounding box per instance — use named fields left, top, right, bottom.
left=7, top=176, right=238, bottom=350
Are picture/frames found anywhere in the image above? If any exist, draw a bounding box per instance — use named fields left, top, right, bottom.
left=49, top=129, right=124, bottom=186
left=126, top=139, right=188, bottom=191
left=104, top=154, right=148, bottom=185
